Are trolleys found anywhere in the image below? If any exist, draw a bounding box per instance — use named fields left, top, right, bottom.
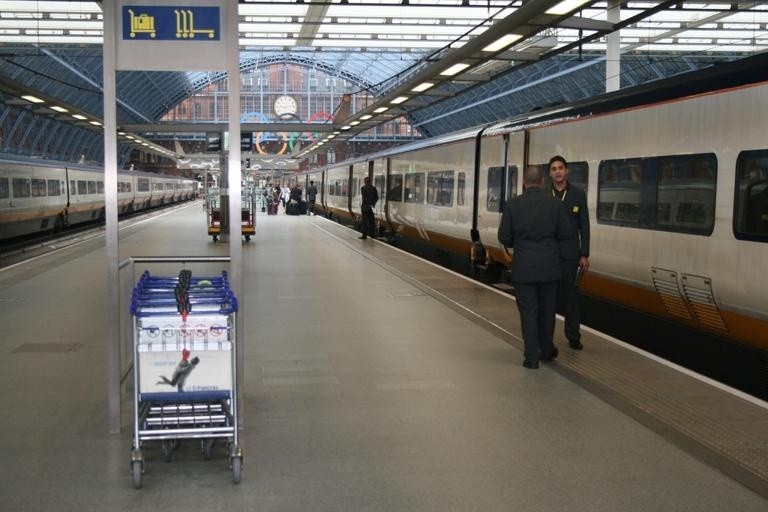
left=205, top=192, right=255, bottom=242
left=129, top=269, right=243, bottom=490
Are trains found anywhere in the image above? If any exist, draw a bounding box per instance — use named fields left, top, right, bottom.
left=276, top=49, right=766, bottom=355
left=1, top=152, right=197, bottom=241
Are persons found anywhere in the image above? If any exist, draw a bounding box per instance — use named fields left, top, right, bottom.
left=307, top=180, right=318, bottom=215
left=540, top=156, right=591, bottom=350
left=358, top=177, right=378, bottom=239
left=266, top=182, right=291, bottom=210
left=157, top=350, right=200, bottom=392
left=498, top=166, right=573, bottom=369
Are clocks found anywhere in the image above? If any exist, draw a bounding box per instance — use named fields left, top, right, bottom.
left=272, top=94, right=298, bottom=120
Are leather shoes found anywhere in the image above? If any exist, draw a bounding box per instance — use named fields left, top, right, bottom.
left=523, top=359, right=538, bottom=369
left=569, top=337, right=583, bottom=350
left=543, top=347, right=558, bottom=362
